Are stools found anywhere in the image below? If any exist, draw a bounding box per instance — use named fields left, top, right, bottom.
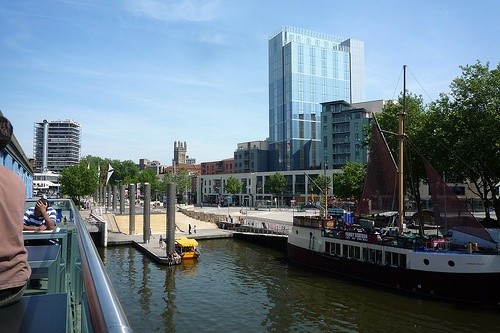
left=23, top=259, right=65, bottom=294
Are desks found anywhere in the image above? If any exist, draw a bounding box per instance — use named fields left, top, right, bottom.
left=0, top=228, right=74, bottom=333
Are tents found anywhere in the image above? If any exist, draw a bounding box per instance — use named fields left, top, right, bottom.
left=176, top=238, right=199, bottom=253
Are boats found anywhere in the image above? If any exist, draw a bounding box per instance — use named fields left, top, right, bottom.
left=174, top=237, right=200, bottom=259
left=286, top=210, right=500, bottom=308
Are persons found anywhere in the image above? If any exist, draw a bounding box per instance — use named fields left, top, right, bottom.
left=188, top=224, right=192, bottom=235
left=193, top=225, right=197, bottom=234
left=150, top=227, right=155, bottom=239
left=159, top=235, right=164, bottom=250
left=200, top=200, right=235, bottom=209
left=22, top=198, right=57, bottom=290
left=0, top=116, right=31, bottom=307
left=82, top=200, right=91, bottom=210
